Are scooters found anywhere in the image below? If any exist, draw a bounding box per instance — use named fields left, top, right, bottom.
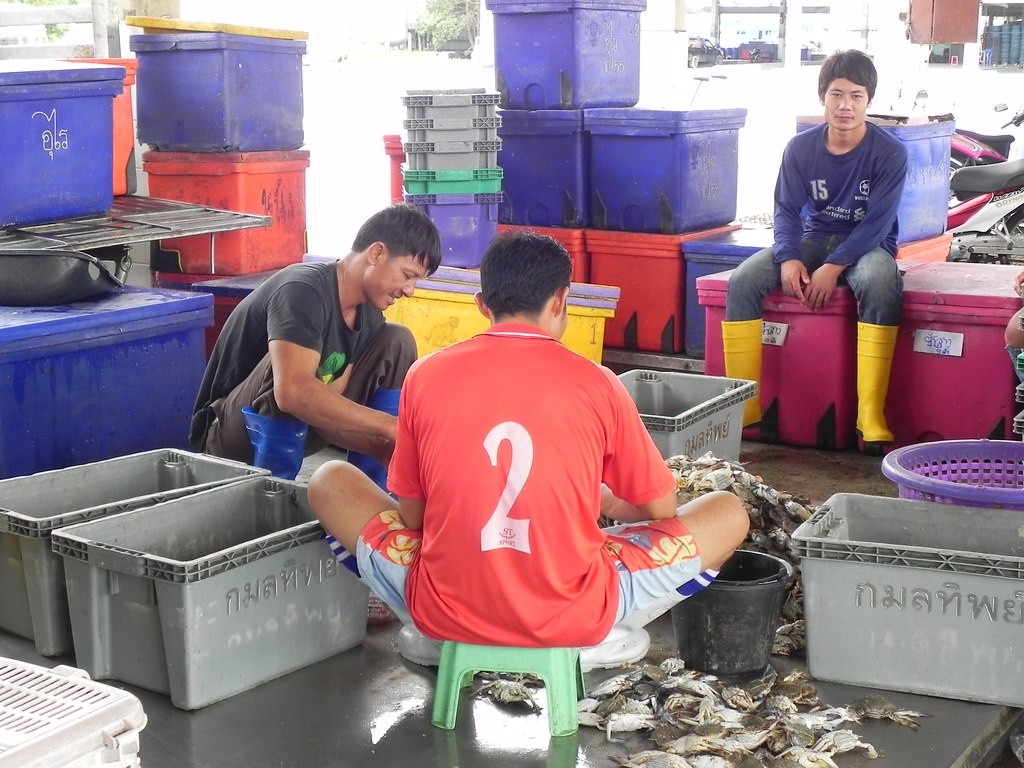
left=950, top=110, right=1024, bottom=264
left=749, top=48, right=763, bottom=63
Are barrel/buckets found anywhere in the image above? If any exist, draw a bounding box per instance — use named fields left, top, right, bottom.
left=671, top=550, right=795, bottom=680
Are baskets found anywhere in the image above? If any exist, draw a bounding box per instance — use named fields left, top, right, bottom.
left=881, top=439, right=1024, bottom=510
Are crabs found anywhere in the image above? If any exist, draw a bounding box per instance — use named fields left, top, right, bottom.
left=470, top=450, right=937, bottom=768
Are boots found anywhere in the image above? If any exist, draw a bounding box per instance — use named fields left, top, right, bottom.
left=857, top=322, right=900, bottom=445
left=347, top=389, right=400, bottom=501
left=581, top=588, right=692, bottom=674
left=722, top=317, right=762, bottom=431
left=357, top=579, right=443, bottom=666
left=242, top=406, right=308, bottom=481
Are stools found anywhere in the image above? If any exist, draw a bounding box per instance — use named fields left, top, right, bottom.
left=429, top=640, right=586, bottom=737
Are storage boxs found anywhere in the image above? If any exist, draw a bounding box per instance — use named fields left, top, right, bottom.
left=0, top=1, right=1024, bottom=471
left=0, top=443, right=272, bottom=655
left=791, top=489, right=1024, bottom=714
left=48, top=478, right=371, bottom=713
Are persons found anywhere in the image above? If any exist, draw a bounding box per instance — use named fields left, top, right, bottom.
left=307, top=227, right=750, bottom=672
left=720, top=49, right=904, bottom=442
left=1004, top=270, right=1024, bottom=382
left=188, top=202, right=441, bottom=497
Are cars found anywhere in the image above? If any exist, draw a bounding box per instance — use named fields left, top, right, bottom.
left=687, top=38, right=727, bottom=69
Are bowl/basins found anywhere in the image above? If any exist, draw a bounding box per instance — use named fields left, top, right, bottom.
left=992, top=26, right=1002, bottom=38
